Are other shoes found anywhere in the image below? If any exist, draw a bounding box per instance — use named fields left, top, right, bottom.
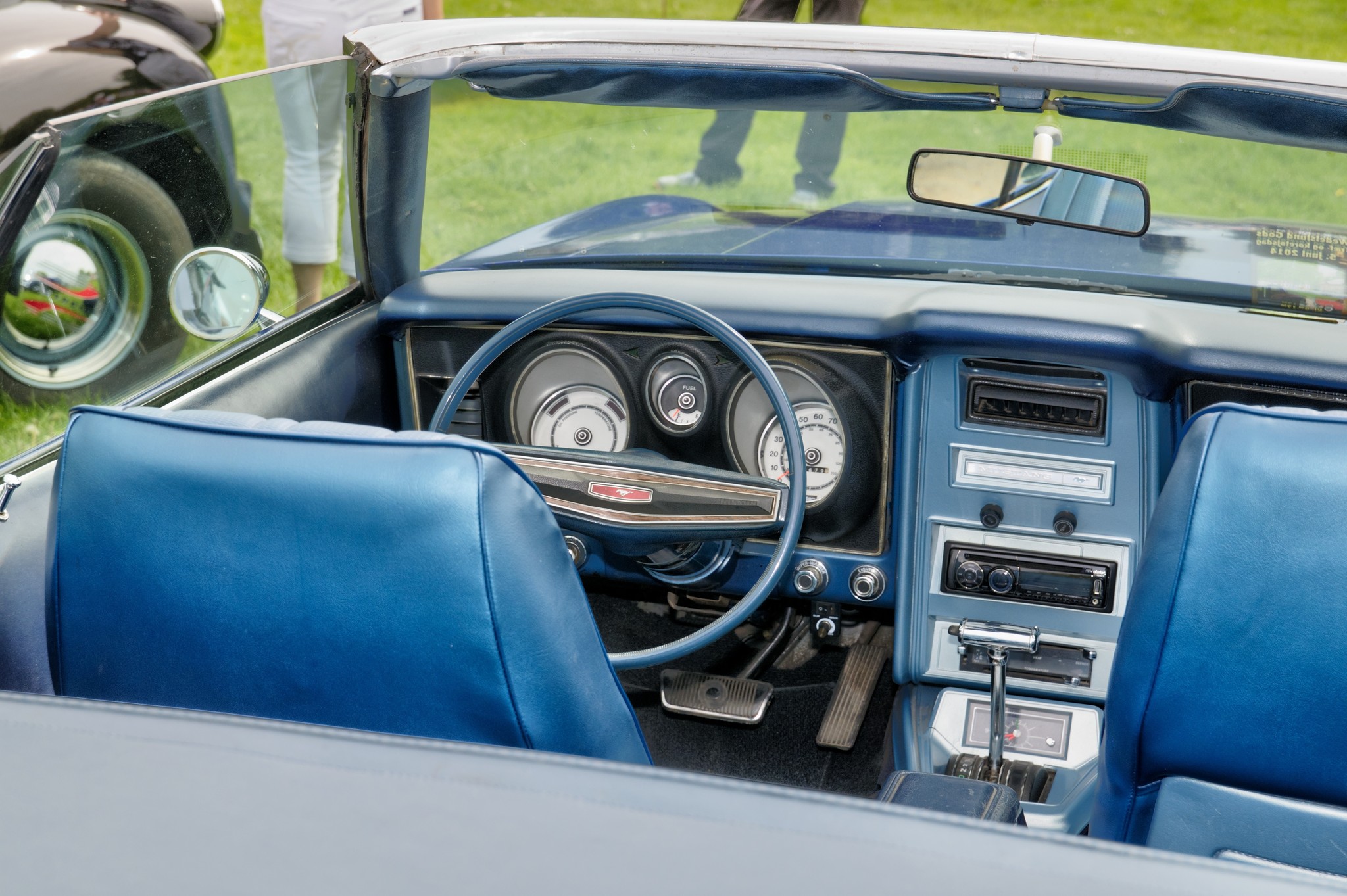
left=792, top=189, right=820, bottom=207
left=660, top=168, right=734, bottom=187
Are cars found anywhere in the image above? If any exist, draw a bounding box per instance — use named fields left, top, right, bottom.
left=20, top=272, right=61, bottom=296
left=0, top=0, right=266, bottom=412
left=0, top=20, right=1347, bottom=895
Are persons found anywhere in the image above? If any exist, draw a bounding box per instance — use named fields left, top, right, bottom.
left=261, top=1, right=447, bottom=317
left=652, top=1, right=870, bottom=210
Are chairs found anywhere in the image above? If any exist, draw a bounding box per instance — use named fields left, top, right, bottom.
left=1090, top=403, right=1347, bottom=880
left=51, top=403, right=655, bottom=765
